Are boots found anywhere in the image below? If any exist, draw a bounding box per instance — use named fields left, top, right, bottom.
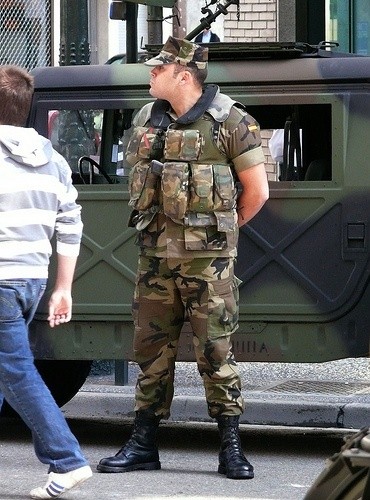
left=97, top=410, right=162, bottom=472
left=217, top=415, right=254, bottom=480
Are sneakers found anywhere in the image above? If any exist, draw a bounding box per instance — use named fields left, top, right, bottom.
left=29, top=465, right=93, bottom=500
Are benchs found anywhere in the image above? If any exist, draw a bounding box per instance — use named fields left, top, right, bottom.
left=303, top=159, right=332, bottom=181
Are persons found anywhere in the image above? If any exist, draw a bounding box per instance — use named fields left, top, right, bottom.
left=1, top=64, right=93, bottom=500
left=269, top=129, right=302, bottom=181
left=97, top=36, right=269, bottom=480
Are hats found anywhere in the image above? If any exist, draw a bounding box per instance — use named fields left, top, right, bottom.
left=144, top=35, right=209, bottom=69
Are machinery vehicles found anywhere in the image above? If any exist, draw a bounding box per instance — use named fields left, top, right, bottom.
left=303, top=427, right=369, bottom=498
left=1, top=1, right=369, bottom=418
